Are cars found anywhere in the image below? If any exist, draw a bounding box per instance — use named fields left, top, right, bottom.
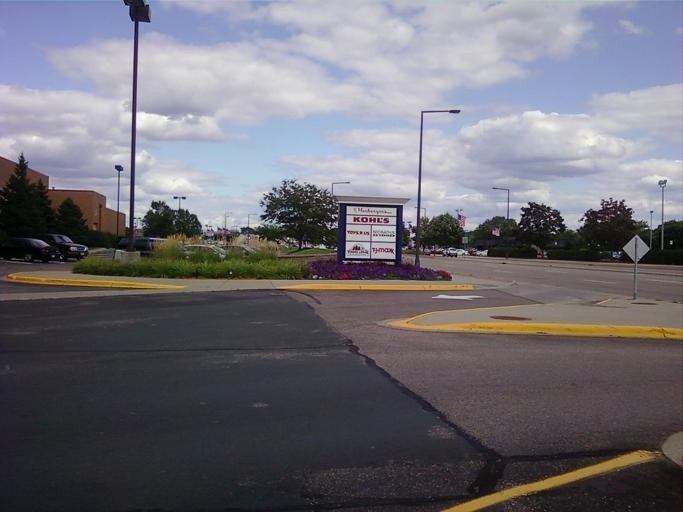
left=400, top=246, right=488, bottom=257
left=600, top=251, right=622, bottom=261
left=0, top=233, right=88, bottom=262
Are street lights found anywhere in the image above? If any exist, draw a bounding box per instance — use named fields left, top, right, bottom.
left=331, top=182, right=350, bottom=196
left=658, top=179, right=667, bottom=250
left=493, top=188, right=509, bottom=220
left=414, top=111, right=460, bottom=267
left=173, top=196, right=186, bottom=211
left=247, top=213, right=258, bottom=245
left=649, top=210, right=653, bottom=250
left=115, top=0, right=151, bottom=251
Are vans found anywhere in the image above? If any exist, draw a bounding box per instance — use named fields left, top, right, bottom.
left=112, top=237, right=256, bottom=260
